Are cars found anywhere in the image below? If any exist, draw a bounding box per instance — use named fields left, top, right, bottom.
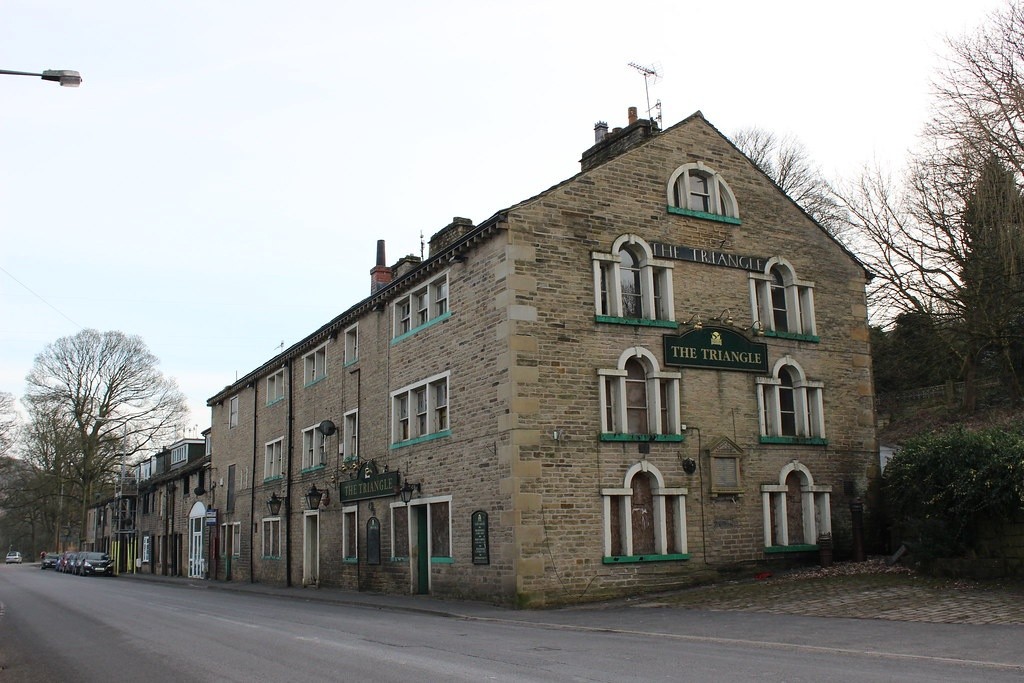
left=56, top=551, right=115, bottom=576
left=6, top=551, right=23, bottom=564
left=41, top=552, right=59, bottom=570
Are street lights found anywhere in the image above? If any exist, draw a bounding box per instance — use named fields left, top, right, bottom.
left=93, top=416, right=127, bottom=576
left=45, top=473, right=63, bottom=552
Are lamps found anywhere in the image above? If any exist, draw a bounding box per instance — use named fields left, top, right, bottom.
left=331, top=454, right=389, bottom=482
left=266, top=491, right=291, bottom=516
left=399, top=477, right=422, bottom=504
left=681, top=308, right=765, bottom=337
left=305, top=483, right=330, bottom=510
